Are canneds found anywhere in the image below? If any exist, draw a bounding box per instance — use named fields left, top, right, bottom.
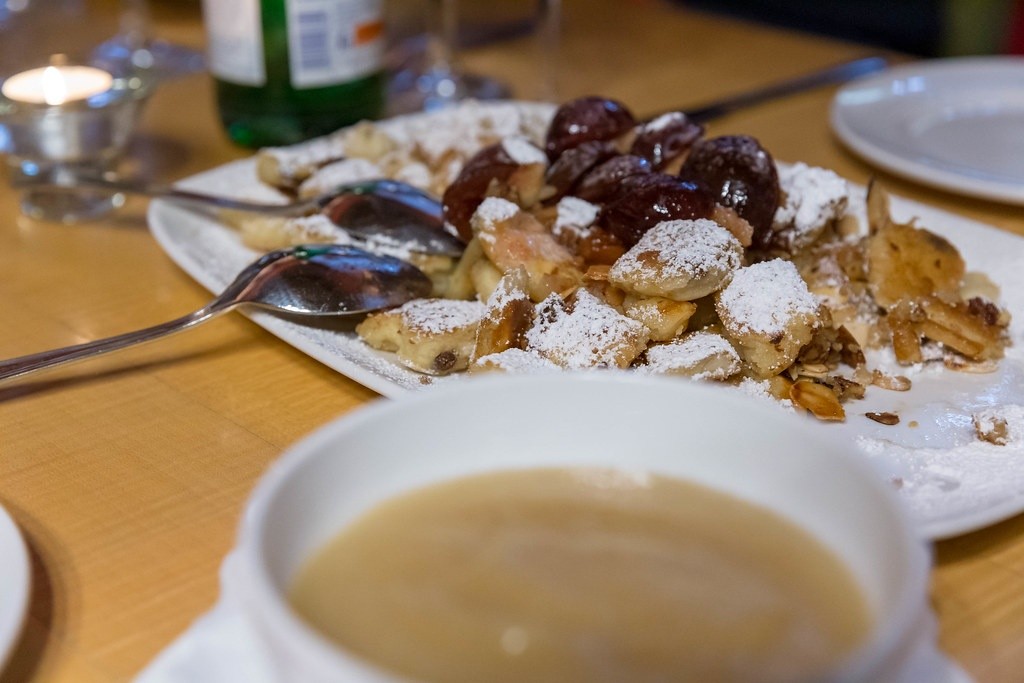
left=195, top=1, right=384, bottom=148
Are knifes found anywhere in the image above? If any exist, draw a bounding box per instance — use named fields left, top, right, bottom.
left=638, top=55, right=883, bottom=125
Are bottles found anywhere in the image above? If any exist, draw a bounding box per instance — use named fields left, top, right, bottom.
left=200, top=0, right=393, bottom=148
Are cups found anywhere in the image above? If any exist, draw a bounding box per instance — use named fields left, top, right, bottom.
left=366, top=1, right=564, bottom=124
left=2, top=1, right=160, bottom=223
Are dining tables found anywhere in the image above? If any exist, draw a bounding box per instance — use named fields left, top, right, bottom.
left=2, top=0, right=1021, bottom=682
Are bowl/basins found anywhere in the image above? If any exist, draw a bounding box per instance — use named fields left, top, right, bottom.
left=223, top=365, right=938, bottom=683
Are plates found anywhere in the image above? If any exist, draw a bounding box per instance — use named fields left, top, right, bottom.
left=127, top=590, right=984, bottom=683
left=0, top=493, right=39, bottom=681
left=831, top=52, right=1023, bottom=208
left=148, top=98, right=1022, bottom=545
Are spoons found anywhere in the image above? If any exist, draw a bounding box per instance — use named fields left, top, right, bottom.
left=2, top=242, right=432, bottom=383
left=9, top=157, right=467, bottom=263
